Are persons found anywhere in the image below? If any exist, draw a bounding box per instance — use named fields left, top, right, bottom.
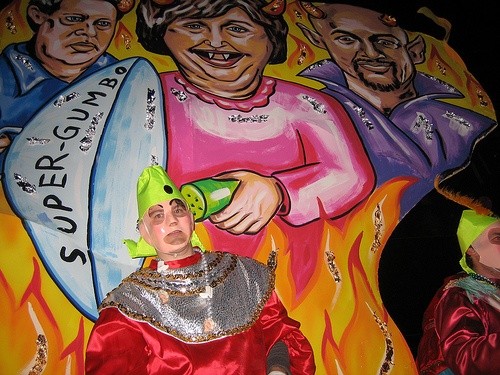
left=415, top=210, right=499, bottom=375
left=84, top=164, right=317, bottom=375
